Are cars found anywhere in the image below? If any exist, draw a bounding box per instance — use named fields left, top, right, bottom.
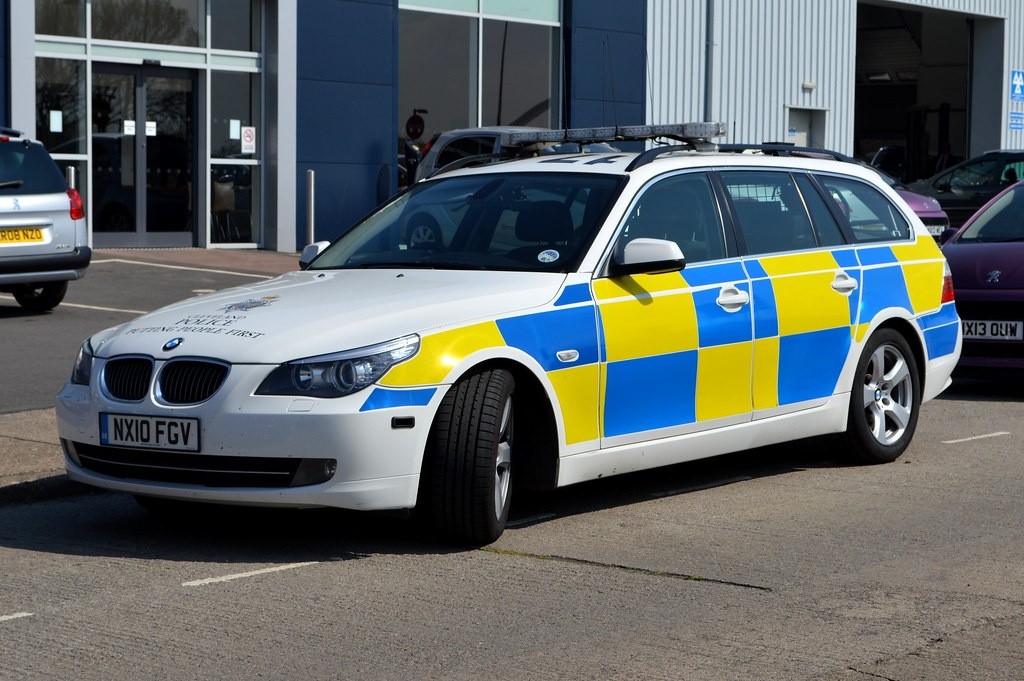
left=938, top=181, right=1024, bottom=391
left=57, top=122, right=964, bottom=548
left=833, top=162, right=952, bottom=241
left=414, top=125, right=623, bottom=253
left=908, top=148, right=1024, bottom=230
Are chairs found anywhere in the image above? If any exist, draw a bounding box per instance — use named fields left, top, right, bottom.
left=508, top=199, right=580, bottom=273
left=639, top=186, right=704, bottom=263
left=1002, top=168, right=1018, bottom=187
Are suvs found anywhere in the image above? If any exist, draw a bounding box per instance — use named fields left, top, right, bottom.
left=0, top=127, right=92, bottom=316
